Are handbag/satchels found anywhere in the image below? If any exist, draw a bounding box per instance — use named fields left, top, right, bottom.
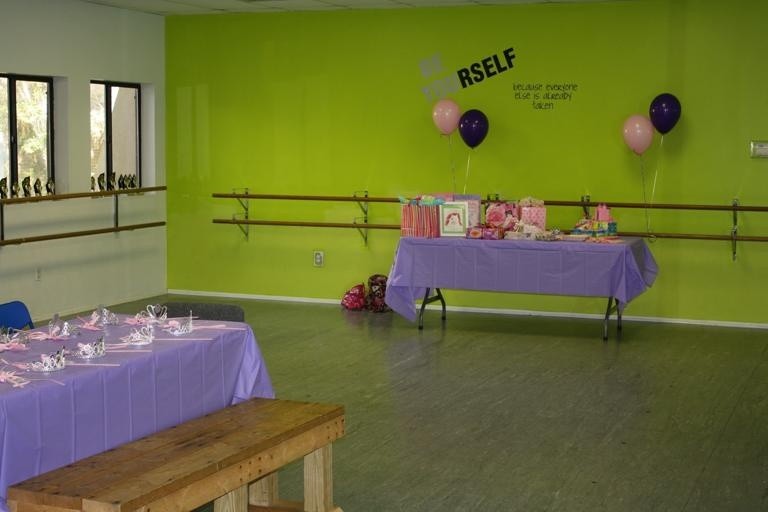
left=340, top=283, right=365, bottom=312
left=366, top=274, right=393, bottom=314
left=400, top=202, right=440, bottom=239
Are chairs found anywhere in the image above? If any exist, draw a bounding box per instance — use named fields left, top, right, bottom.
left=0, top=301, right=34, bottom=333
left=161, top=303, right=244, bottom=322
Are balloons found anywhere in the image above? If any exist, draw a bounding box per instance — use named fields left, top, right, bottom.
left=431, top=99, right=462, bottom=136
left=649, top=91, right=682, bottom=135
left=458, top=108, right=489, bottom=150
left=622, top=114, right=654, bottom=156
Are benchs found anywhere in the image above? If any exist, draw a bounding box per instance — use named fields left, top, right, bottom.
left=6, top=398, right=346, bottom=512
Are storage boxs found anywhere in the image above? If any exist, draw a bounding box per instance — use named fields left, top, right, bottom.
left=439, top=200, right=479, bottom=237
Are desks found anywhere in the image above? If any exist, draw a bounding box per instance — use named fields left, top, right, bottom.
left=384, top=237, right=659, bottom=341
left=0, top=313, right=275, bottom=512
